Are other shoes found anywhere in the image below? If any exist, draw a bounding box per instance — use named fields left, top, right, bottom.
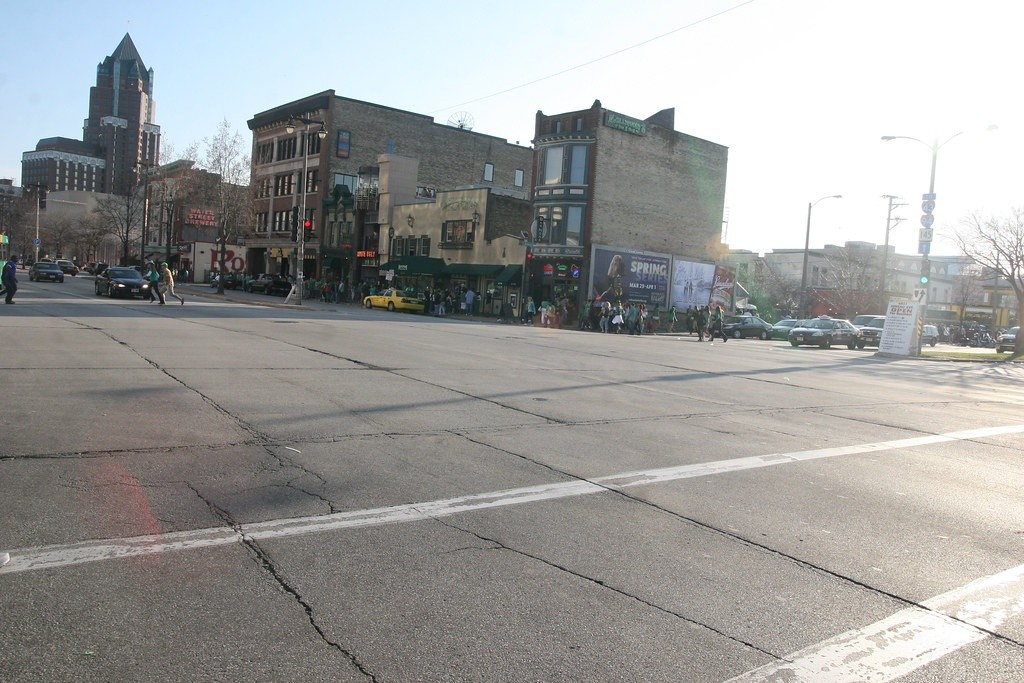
left=181, top=297, right=184, bottom=305
left=150, top=298, right=157, bottom=303
left=698, top=339, right=703, bottom=342
left=708, top=338, right=713, bottom=341
left=724, top=336, right=728, bottom=342
left=158, top=302, right=166, bottom=305
left=6, top=301, right=15, bottom=304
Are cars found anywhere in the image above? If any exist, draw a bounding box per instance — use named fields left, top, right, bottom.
left=17, top=255, right=109, bottom=277
left=787, top=313, right=860, bottom=350
left=921, top=324, right=940, bottom=347
left=768, top=318, right=799, bottom=340
left=94, top=267, right=151, bottom=300
left=713, top=315, right=774, bottom=341
left=363, top=287, right=426, bottom=314
left=28, top=261, right=64, bottom=283
left=210, top=274, right=237, bottom=290
left=850, top=314, right=887, bottom=349
left=996, top=326, right=1021, bottom=354
left=246, top=272, right=293, bottom=297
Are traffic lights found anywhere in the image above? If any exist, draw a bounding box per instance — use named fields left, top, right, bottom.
left=920, top=259, right=930, bottom=285
left=304, top=220, right=311, bottom=242
left=524, top=246, right=533, bottom=273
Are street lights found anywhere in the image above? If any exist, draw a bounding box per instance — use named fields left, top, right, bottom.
left=796, top=194, right=842, bottom=319
left=131, top=161, right=149, bottom=274
left=150, top=163, right=164, bottom=246
left=285, top=111, right=330, bottom=306
left=25, top=181, right=51, bottom=263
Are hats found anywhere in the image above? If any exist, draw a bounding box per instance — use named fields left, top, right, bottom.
left=145, top=261, right=155, bottom=265
left=160, top=262, right=168, bottom=268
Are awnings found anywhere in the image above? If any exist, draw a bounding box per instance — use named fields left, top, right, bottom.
left=136, top=252, right=177, bottom=262
left=379, top=257, right=523, bottom=286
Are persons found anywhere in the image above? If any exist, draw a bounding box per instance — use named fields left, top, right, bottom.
left=971, top=320, right=980, bottom=329
left=577, top=297, right=728, bottom=342
left=526, top=291, right=575, bottom=327
left=143, top=260, right=185, bottom=305
left=735, top=308, right=759, bottom=339
left=0, top=255, right=19, bottom=304
left=210, top=268, right=478, bottom=318
left=929, top=321, right=966, bottom=345
